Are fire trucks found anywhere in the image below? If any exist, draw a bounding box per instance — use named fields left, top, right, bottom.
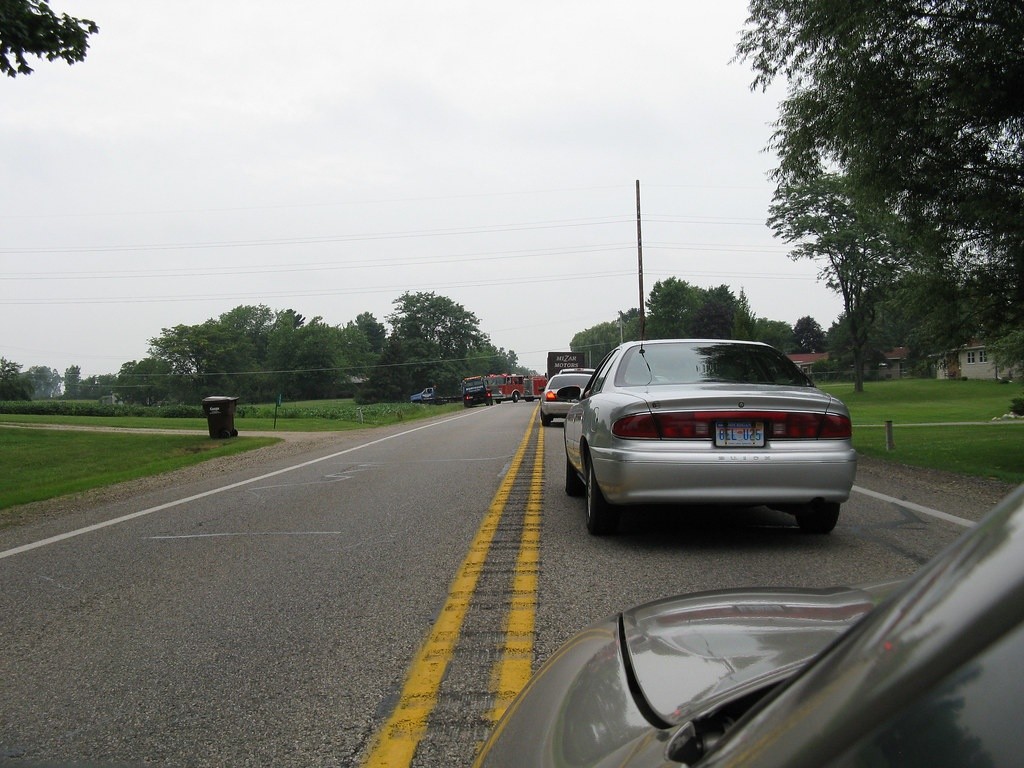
left=489, top=374, right=546, bottom=404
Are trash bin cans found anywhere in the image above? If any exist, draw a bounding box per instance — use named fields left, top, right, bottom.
left=203, top=396, right=240, bottom=438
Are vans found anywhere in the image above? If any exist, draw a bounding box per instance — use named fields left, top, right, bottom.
left=558, top=368, right=596, bottom=374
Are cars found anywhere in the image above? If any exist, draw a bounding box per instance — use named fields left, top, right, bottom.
left=467, top=471, right=1024, bottom=768
left=565, top=340, right=857, bottom=538
left=540, top=374, right=593, bottom=426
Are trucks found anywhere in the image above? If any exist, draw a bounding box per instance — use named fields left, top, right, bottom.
left=546, top=351, right=586, bottom=381
left=409, top=387, right=464, bottom=405
left=462, top=378, right=494, bottom=407
